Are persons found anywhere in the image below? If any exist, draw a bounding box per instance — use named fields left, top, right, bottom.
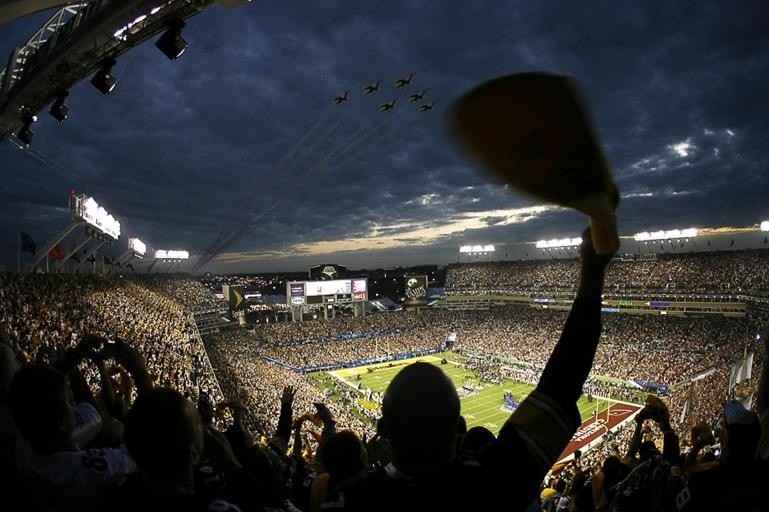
left=317, top=215, right=621, bottom=510
left=0, top=249, right=769, bottom=512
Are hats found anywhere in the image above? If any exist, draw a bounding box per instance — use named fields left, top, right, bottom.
left=725, top=401, right=760, bottom=455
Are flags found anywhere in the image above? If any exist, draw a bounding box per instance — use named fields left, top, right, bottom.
left=19, top=233, right=136, bottom=272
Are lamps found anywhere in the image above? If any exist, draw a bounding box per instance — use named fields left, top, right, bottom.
left=0, top=8, right=202, bottom=149
